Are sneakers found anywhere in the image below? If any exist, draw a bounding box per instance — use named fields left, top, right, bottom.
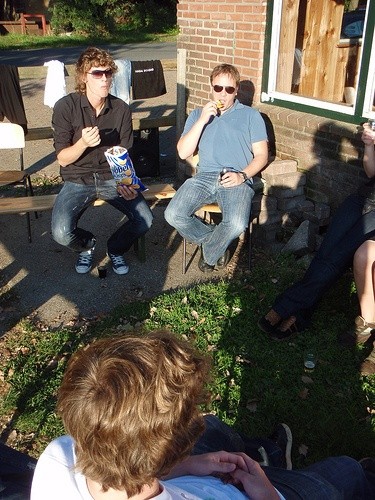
left=74, top=237, right=97, bottom=273
left=108, top=248, right=129, bottom=274
left=335, top=315, right=375, bottom=345
left=357, top=341, right=375, bottom=374
left=258, top=308, right=282, bottom=333
left=359, top=457, right=375, bottom=473
left=267, top=423, right=293, bottom=470
left=270, top=315, right=305, bottom=340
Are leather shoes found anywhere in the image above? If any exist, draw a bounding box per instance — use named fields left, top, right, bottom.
left=199, top=243, right=215, bottom=271
left=216, top=247, right=231, bottom=268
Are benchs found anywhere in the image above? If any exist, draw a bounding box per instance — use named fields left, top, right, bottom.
left=0, top=183, right=177, bottom=264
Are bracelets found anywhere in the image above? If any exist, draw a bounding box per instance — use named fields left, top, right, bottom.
left=238, top=170, right=249, bottom=181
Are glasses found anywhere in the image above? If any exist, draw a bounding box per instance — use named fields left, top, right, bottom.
left=211, top=84, right=238, bottom=94
left=86, top=68, right=113, bottom=79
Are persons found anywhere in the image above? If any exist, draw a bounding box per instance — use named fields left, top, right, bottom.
left=163, top=62, right=270, bottom=273
left=336, top=236, right=375, bottom=379
left=255, top=120, right=375, bottom=344
left=28, top=324, right=375, bottom=500
left=50, top=45, right=154, bottom=275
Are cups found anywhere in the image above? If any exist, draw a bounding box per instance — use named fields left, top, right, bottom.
left=304, top=358, right=316, bottom=373
left=97, top=266, right=107, bottom=278
left=218, top=168, right=231, bottom=185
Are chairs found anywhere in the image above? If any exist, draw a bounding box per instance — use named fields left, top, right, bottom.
left=0, top=123, right=38, bottom=243
left=182, top=148, right=253, bottom=275
